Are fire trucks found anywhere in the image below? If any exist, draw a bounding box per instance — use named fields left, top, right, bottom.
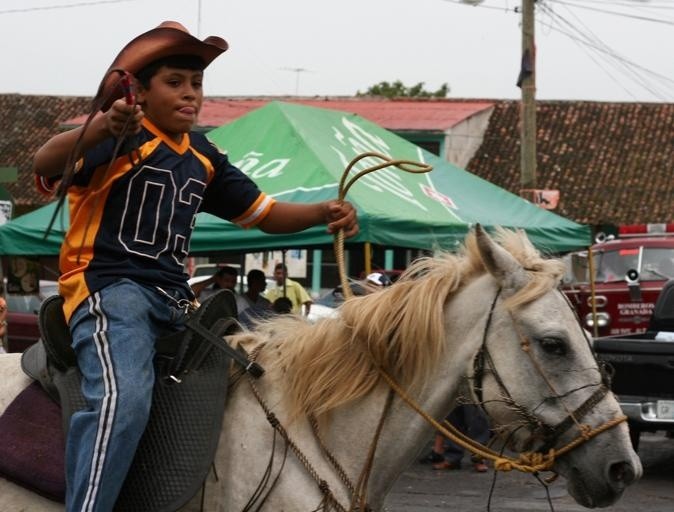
left=561, top=222, right=674, bottom=335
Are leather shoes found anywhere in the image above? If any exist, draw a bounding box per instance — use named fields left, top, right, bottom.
left=433, top=459, right=450, bottom=469
left=473, top=463, right=488, bottom=472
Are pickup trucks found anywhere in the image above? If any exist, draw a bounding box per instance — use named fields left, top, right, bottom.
left=591, top=277, right=674, bottom=453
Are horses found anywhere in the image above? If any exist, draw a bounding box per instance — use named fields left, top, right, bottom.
left=0, top=223, right=645, bottom=512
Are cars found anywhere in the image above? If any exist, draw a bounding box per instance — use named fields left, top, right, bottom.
left=301, top=269, right=406, bottom=324
left=2, top=276, right=59, bottom=353
left=187, top=263, right=275, bottom=300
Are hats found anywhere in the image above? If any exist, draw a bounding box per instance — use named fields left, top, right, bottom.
left=94, top=21, right=229, bottom=113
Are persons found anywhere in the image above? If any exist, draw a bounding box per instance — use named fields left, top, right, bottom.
left=428, top=404, right=494, bottom=473
left=34, top=21, right=358, bottom=512
left=240, top=298, right=298, bottom=323
left=267, top=263, right=312, bottom=312
left=192, top=263, right=248, bottom=313
left=240, top=268, right=269, bottom=313
